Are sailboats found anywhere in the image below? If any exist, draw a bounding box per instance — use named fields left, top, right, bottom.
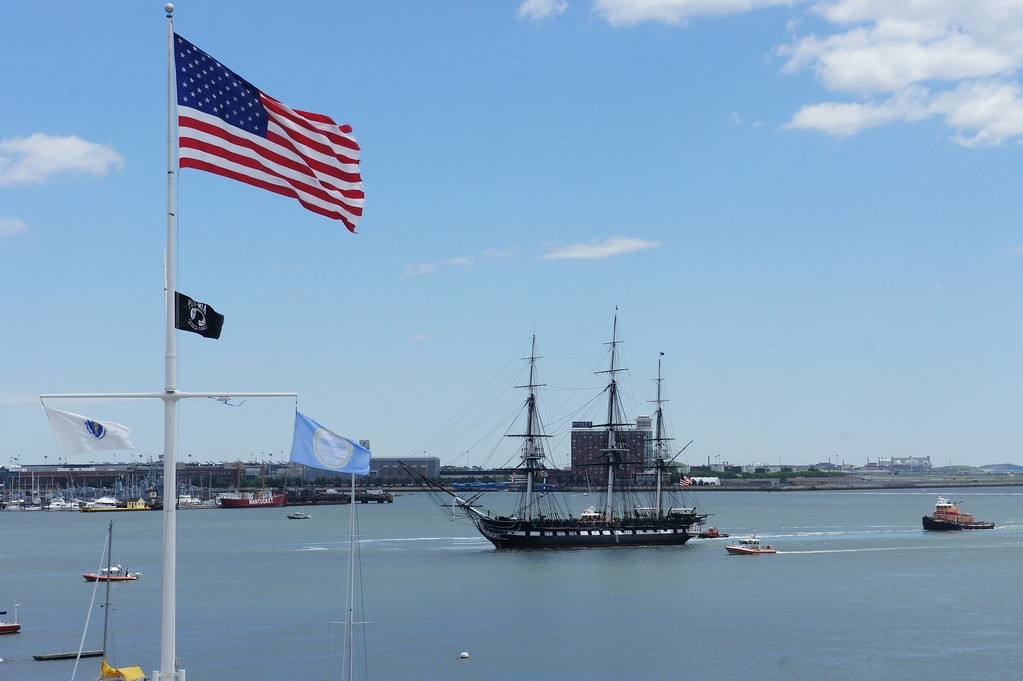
left=395, top=305, right=718, bottom=554
left=71, top=518, right=149, bottom=681
left=3, top=452, right=164, bottom=512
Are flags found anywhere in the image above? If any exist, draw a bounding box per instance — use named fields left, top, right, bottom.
left=684, top=475, right=692, bottom=485
left=512, top=471, right=518, bottom=483
left=173, top=30, right=366, bottom=234
left=680, top=479, right=689, bottom=487
left=47, top=406, right=135, bottom=456
left=173, top=289, right=224, bottom=341
left=290, top=412, right=371, bottom=477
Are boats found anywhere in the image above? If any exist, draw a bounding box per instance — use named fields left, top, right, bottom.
left=724, top=529, right=777, bottom=556
left=175, top=451, right=396, bottom=509
left=0, top=604, right=22, bottom=634
left=921, top=495, right=996, bottom=530
left=82, top=561, right=143, bottom=581
left=286, top=511, right=310, bottom=520
left=697, top=526, right=729, bottom=538
